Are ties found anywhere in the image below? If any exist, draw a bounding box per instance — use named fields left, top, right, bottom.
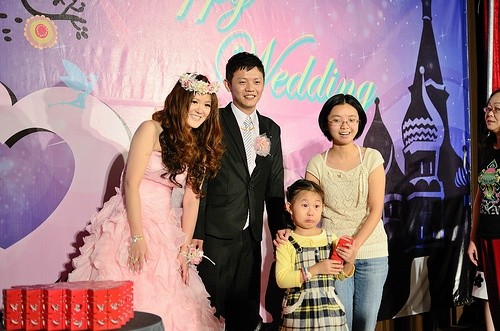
left=243, top=115, right=254, bottom=176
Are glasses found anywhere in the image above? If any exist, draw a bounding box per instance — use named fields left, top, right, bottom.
left=328, top=117, right=360, bottom=126
left=483, top=106, right=500, bottom=113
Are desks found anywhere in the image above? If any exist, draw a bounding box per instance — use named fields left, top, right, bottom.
left=0, top=308, right=166, bottom=331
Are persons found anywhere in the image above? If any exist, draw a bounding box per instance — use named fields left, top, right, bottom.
left=272, top=93, right=389, bottom=331
left=65, top=72, right=224, bottom=331
left=468, top=89, right=500, bottom=330
left=193, top=53, right=296, bottom=331
left=275, top=180, right=357, bottom=331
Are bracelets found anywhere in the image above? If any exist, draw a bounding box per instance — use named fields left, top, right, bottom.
left=300, top=267, right=312, bottom=283
left=177, top=245, right=215, bottom=267
left=130, top=235, right=143, bottom=244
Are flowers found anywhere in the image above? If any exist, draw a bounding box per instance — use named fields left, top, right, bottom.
left=252, top=133, right=273, bottom=158
left=179, top=73, right=220, bottom=95
